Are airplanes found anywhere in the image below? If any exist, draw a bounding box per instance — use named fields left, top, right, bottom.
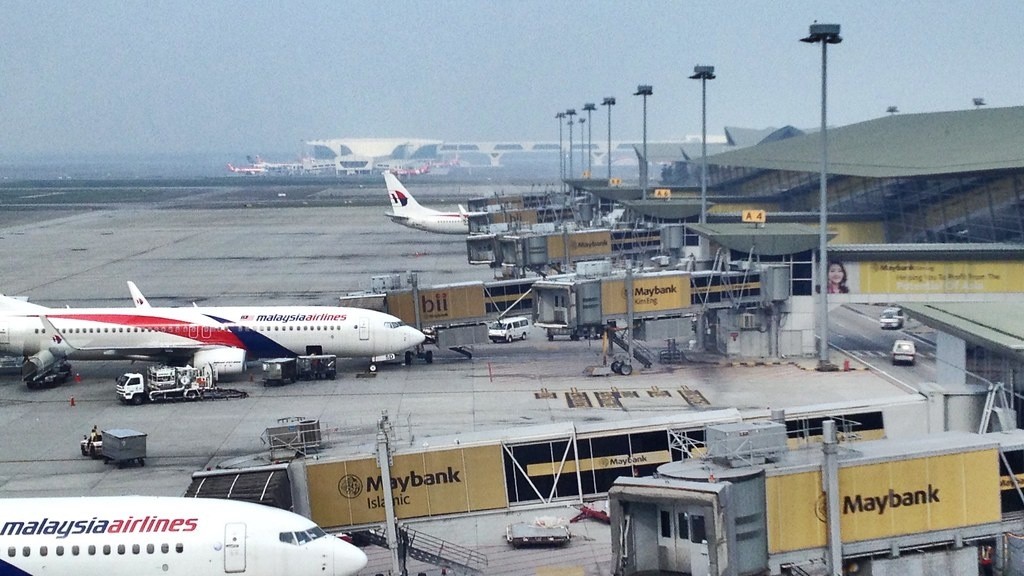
left=381, top=169, right=627, bottom=239
left=0, top=496, right=369, bottom=576
left=1, top=279, right=427, bottom=384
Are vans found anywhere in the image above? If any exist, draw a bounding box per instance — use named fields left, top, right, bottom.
left=881, top=306, right=904, bottom=330
left=489, top=316, right=531, bottom=343
left=891, top=339, right=917, bottom=366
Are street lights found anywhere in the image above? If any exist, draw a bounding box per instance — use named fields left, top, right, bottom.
left=554, top=111, right=568, bottom=174
left=634, top=84, right=654, bottom=199
left=566, top=106, right=577, bottom=177
left=799, top=20, right=840, bottom=370
left=690, top=64, right=716, bottom=270
left=582, top=102, right=597, bottom=174
left=600, top=96, right=616, bottom=180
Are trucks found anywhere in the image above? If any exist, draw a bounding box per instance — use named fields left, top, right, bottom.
left=115, top=361, right=219, bottom=405
